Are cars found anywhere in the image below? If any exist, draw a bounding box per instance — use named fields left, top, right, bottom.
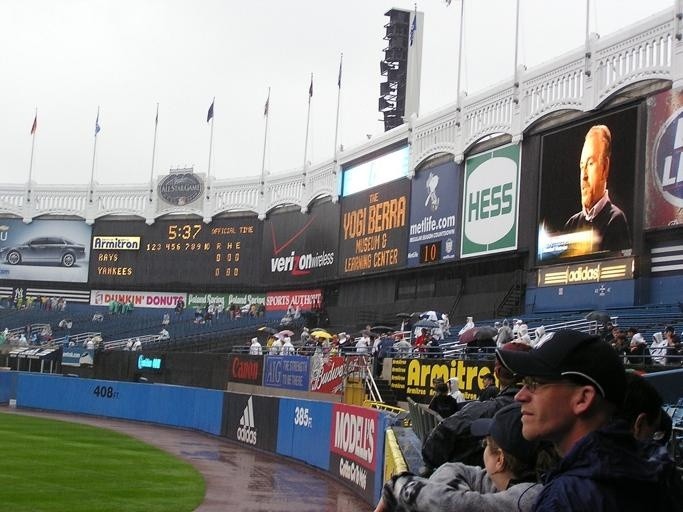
left=3, top=236, right=87, bottom=268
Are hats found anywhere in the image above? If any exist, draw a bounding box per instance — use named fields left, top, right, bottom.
left=494, top=328, right=628, bottom=405
left=469, top=399, right=558, bottom=464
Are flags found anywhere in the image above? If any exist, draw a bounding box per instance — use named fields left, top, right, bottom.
left=264, top=97, right=269, bottom=114
left=207, top=103, right=214, bottom=122
left=95, top=113, right=100, bottom=136
left=337, top=63, right=342, bottom=89
left=31, top=115, right=37, bottom=134
left=156, top=107, right=158, bottom=125
left=309, top=77, right=313, bottom=97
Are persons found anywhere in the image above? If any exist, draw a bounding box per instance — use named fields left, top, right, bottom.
left=562, top=125, right=631, bottom=250
left=1, top=296, right=185, bottom=347
